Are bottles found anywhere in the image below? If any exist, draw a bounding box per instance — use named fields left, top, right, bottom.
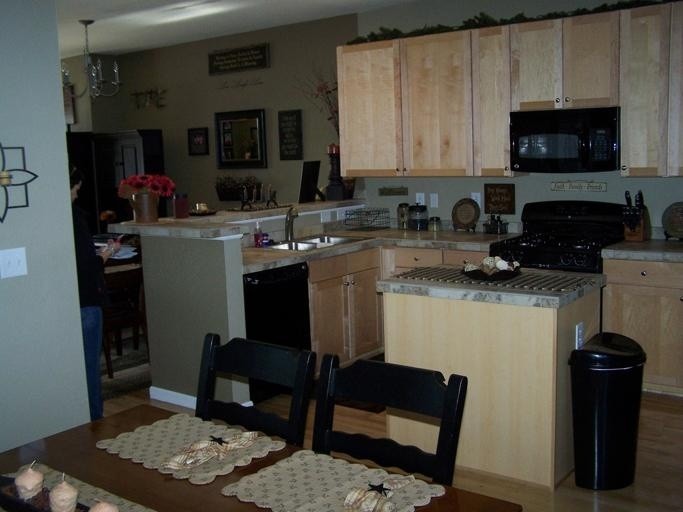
left=172, top=193, right=188, bottom=218
left=396, top=203, right=441, bottom=232
left=483, top=215, right=508, bottom=233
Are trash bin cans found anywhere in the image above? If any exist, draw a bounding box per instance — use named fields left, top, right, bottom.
left=568, top=332, right=646, bottom=491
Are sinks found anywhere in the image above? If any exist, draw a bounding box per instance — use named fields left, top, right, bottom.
left=269, top=242, right=317, bottom=252
left=301, top=234, right=369, bottom=248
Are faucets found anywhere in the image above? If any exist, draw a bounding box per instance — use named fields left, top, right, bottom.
left=284, top=208, right=301, bottom=242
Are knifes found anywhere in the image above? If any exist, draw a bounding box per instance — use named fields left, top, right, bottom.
left=623, top=189, right=647, bottom=224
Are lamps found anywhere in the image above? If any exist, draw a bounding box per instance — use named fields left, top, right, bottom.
left=61, top=19, right=124, bottom=99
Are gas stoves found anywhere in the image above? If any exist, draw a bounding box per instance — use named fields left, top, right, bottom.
left=489, top=234, right=625, bottom=274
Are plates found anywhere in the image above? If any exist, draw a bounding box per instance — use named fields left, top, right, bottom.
left=94, top=241, right=138, bottom=259
left=662, top=202, right=682, bottom=238
left=189, top=208, right=218, bottom=216
left=452, top=198, right=480, bottom=228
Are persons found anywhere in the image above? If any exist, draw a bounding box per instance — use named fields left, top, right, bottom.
left=69, top=166, right=120, bottom=420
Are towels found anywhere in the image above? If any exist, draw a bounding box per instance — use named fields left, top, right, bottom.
left=343, top=475, right=416, bottom=511
left=158, top=431, right=259, bottom=474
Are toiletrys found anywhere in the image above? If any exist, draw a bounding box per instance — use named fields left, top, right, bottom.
left=254, top=221, right=263, bottom=249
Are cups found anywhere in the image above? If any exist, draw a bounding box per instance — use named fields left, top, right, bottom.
left=192, top=200, right=208, bottom=213
left=343, top=208, right=390, bottom=229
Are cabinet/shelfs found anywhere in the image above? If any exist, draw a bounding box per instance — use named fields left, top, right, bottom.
left=336, top=29, right=473, bottom=177
left=602, top=259, right=683, bottom=398
left=509, top=10, right=620, bottom=112
left=394, top=247, right=489, bottom=275
left=307, top=247, right=385, bottom=381
left=471, top=25, right=530, bottom=177
left=65, top=128, right=175, bottom=308
left=619, top=0, right=683, bottom=178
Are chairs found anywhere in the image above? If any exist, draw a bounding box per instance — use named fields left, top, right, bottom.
left=312, top=354, right=468, bottom=488
left=98, top=263, right=150, bottom=379
left=195, top=333, right=316, bottom=450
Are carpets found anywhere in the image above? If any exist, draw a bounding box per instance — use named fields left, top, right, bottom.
left=99, top=332, right=152, bottom=402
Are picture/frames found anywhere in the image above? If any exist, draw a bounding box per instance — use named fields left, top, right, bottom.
left=188, top=107, right=304, bottom=168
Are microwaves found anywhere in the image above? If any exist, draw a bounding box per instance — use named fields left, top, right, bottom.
left=509, top=106, right=621, bottom=173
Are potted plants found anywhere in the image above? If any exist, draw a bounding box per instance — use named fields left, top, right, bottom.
left=215, top=175, right=263, bottom=200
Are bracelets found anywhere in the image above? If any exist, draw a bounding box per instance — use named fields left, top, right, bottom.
left=101, top=247, right=115, bottom=257
